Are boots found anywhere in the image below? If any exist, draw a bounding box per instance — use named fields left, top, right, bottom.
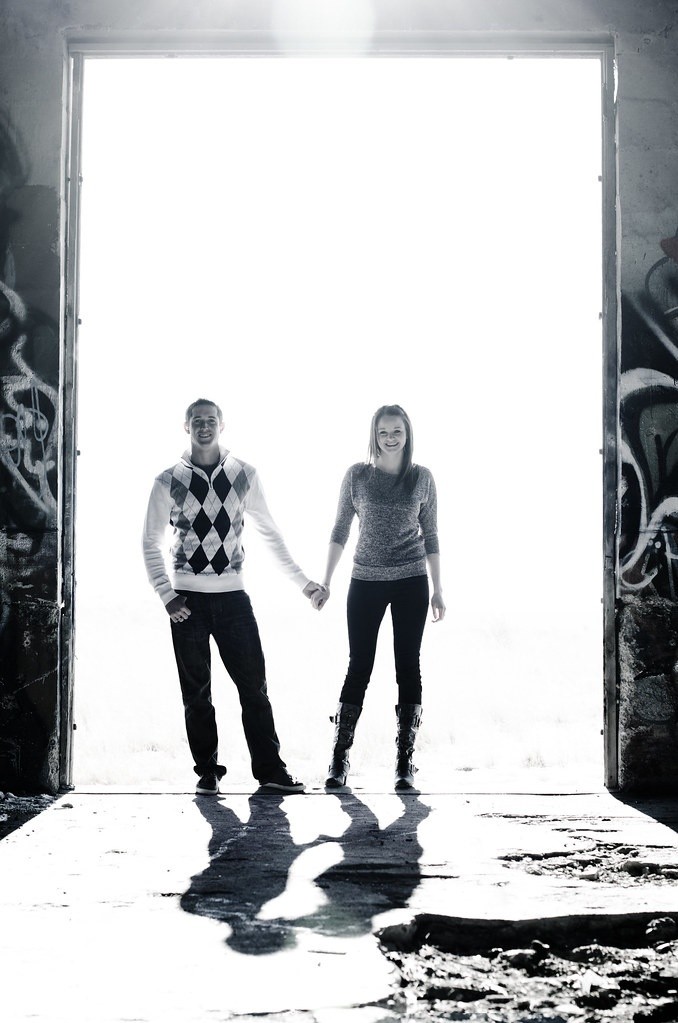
left=395, top=704, right=422, bottom=790
left=325, top=701, right=364, bottom=788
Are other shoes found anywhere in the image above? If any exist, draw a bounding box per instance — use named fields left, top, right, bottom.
left=259, top=764, right=306, bottom=791
left=195, top=774, right=219, bottom=794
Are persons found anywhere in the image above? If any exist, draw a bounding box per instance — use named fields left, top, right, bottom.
left=142, top=399, right=327, bottom=794
left=310, top=405, right=447, bottom=788
